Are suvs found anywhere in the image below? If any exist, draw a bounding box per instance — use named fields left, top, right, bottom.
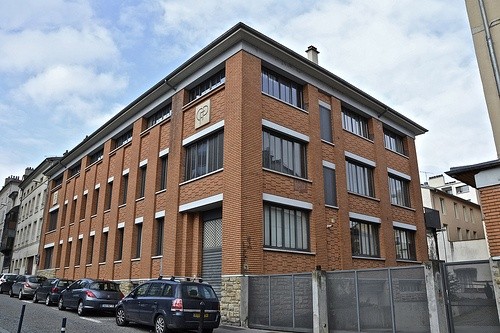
left=115, top=275, right=221, bottom=333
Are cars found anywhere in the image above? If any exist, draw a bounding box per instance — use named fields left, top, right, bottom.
left=32, top=278, right=76, bottom=305
left=9, top=274, right=48, bottom=299
left=0, top=272, right=19, bottom=293
left=57, top=278, right=124, bottom=315
